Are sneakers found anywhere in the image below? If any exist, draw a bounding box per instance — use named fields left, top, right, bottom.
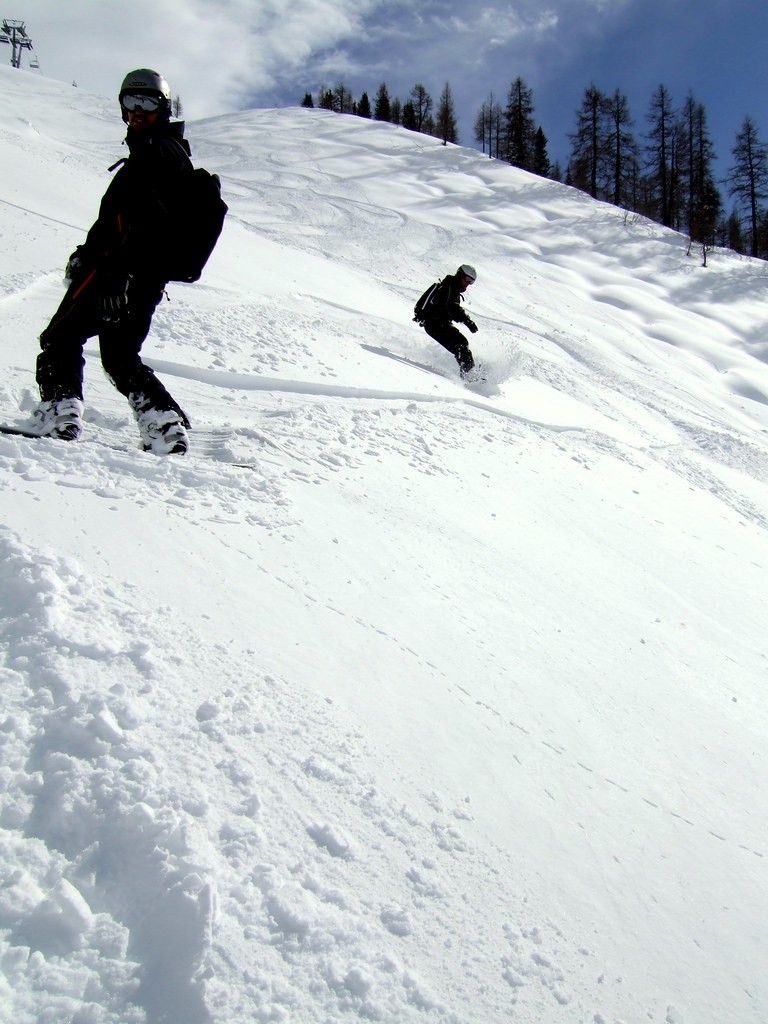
left=137, top=405, right=190, bottom=456
left=0, top=394, right=83, bottom=441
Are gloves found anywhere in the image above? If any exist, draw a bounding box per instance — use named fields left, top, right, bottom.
left=94, top=257, right=134, bottom=323
left=65, top=246, right=89, bottom=279
left=464, top=316, right=478, bottom=333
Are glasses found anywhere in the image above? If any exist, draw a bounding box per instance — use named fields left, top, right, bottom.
left=120, top=92, right=162, bottom=113
left=458, top=267, right=475, bottom=285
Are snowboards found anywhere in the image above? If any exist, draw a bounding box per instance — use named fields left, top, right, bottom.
left=4, top=422, right=255, bottom=471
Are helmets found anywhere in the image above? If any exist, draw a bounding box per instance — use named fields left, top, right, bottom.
left=457, top=265, right=477, bottom=281
left=119, top=69, right=171, bottom=112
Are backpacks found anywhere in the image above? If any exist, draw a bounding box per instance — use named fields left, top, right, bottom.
left=412, top=283, right=441, bottom=327
left=120, top=136, right=228, bottom=284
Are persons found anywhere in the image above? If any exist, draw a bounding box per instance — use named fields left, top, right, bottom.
left=1, top=70, right=188, bottom=457
left=422, top=265, right=477, bottom=381
